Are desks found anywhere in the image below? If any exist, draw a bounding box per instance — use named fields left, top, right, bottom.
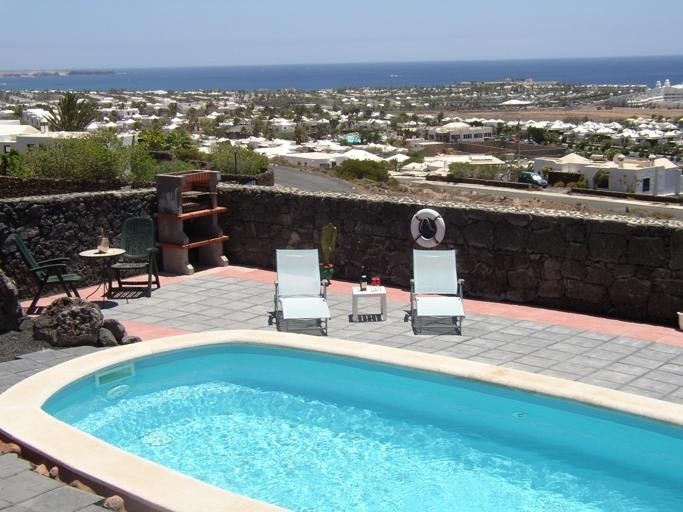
left=77, top=248, right=127, bottom=308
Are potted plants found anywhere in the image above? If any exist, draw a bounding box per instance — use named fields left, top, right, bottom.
left=319, top=222, right=336, bottom=285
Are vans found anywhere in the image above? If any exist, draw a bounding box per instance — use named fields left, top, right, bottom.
left=518, top=172, right=548, bottom=188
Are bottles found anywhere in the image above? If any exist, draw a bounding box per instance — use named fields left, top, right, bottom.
left=360, top=265, right=368, bottom=291
left=98, top=227, right=104, bottom=239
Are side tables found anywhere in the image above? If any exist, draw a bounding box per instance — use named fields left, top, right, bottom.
left=351, top=285, right=387, bottom=322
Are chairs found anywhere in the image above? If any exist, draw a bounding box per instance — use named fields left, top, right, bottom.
left=106, top=214, right=160, bottom=299
left=7, top=233, right=83, bottom=315
left=408, top=248, right=466, bottom=335
left=273, top=248, right=330, bottom=337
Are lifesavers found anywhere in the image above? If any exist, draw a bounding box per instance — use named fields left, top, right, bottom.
left=411, top=208, right=445, bottom=248
